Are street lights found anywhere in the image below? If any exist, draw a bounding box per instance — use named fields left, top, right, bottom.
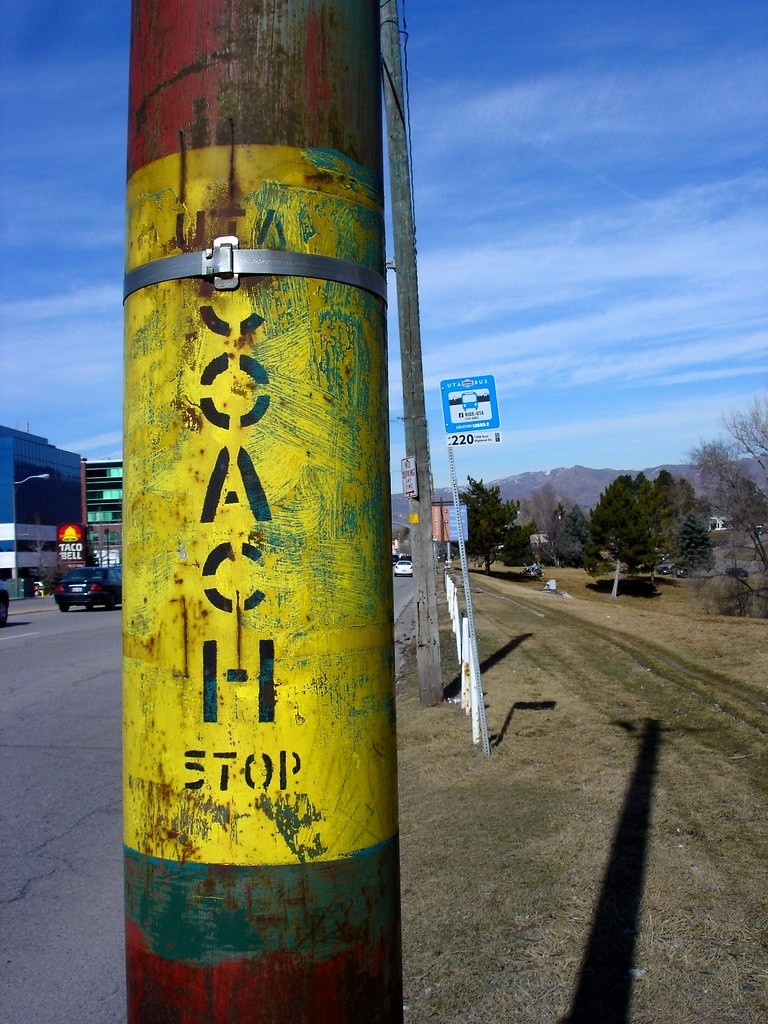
left=13, top=473, right=50, bottom=596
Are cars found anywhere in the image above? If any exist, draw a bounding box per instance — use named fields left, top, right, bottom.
left=54, top=566, right=122, bottom=612
left=393, top=560, right=413, bottom=578
left=0, top=580, right=10, bottom=628
